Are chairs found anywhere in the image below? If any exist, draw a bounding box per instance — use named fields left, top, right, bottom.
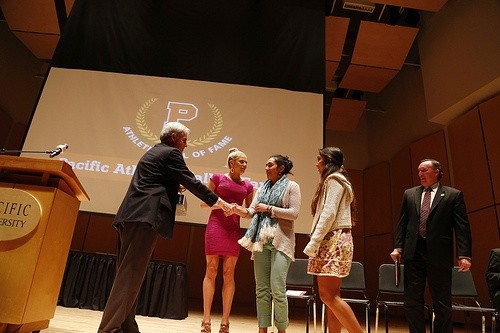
left=270, top=258, right=496, bottom=333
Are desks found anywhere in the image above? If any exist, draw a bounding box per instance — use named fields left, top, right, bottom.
left=56, top=248, right=189, bottom=320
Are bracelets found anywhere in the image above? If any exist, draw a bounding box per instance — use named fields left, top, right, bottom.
left=267, top=205, right=272, bottom=215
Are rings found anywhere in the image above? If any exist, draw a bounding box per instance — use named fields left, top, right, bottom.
left=467, top=268, right=470, bottom=269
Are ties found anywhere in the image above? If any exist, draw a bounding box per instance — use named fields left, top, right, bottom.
left=419, top=188, right=432, bottom=239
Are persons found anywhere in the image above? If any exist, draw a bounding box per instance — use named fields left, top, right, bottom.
left=97, top=122, right=237, bottom=333
left=200, top=148, right=253, bottom=333
left=303, top=146, right=363, bottom=333
left=223, top=154, right=301, bottom=333
left=390, top=159, right=472, bottom=333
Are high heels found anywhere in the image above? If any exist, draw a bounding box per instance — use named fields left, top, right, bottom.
left=201, top=320, right=211, bottom=333
left=219, top=322, right=229, bottom=333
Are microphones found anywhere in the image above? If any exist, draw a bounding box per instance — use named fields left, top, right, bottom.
left=49, top=143, right=69, bottom=158
